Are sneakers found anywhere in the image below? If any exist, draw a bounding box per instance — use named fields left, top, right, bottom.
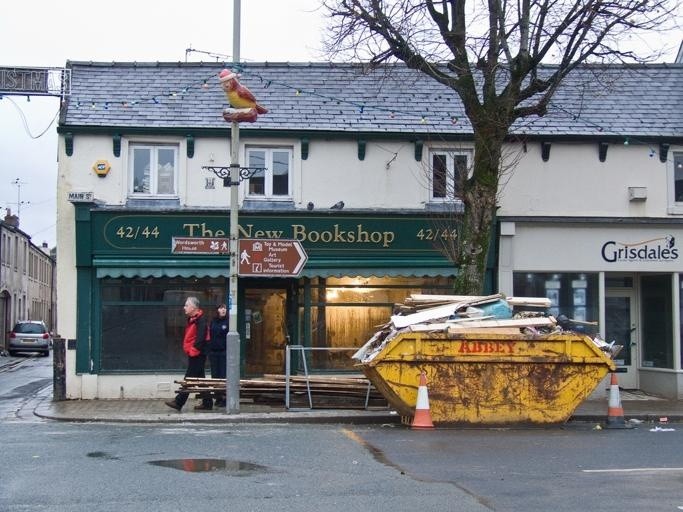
left=194, top=400, right=226, bottom=410
left=165, top=401, right=182, bottom=411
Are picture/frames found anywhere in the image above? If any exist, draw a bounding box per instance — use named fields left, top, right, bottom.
left=253, top=311, right=263, bottom=324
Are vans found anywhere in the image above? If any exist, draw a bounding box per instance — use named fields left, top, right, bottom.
left=162, top=291, right=208, bottom=338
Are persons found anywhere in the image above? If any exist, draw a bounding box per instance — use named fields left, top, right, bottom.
left=206, top=303, right=230, bottom=408
left=162, top=295, right=213, bottom=411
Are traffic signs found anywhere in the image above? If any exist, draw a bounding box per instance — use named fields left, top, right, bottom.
left=236, top=238, right=308, bottom=278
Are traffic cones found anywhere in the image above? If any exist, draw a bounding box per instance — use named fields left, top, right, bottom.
left=411, top=370, right=434, bottom=430
left=607, top=373, right=625, bottom=429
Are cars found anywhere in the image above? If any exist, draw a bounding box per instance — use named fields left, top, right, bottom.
left=9, top=319, right=50, bottom=356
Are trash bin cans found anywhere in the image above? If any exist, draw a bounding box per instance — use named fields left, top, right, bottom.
left=353, top=332, right=618, bottom=427
